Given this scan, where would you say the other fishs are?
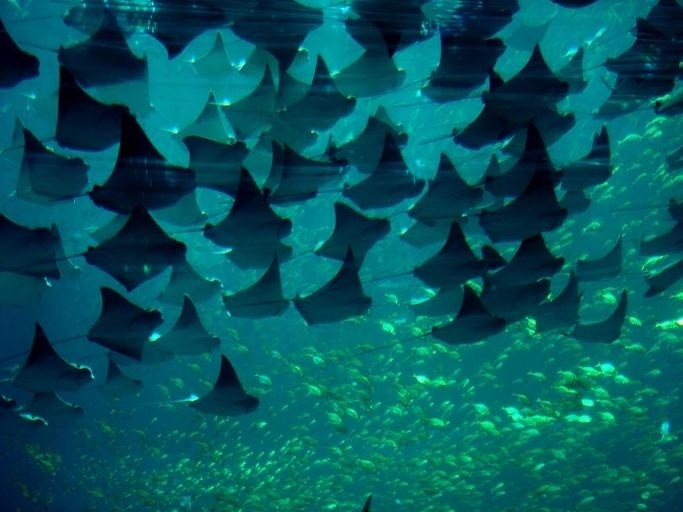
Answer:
[0,0,683,512]
